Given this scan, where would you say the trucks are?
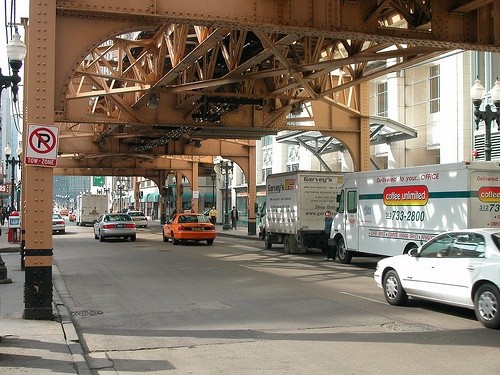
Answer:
[253,170,355,254]
[75,194,108,226]
[329,161,500,264]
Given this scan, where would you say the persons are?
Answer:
[0,205,16,225]
[210,207,217,225]
[324,211,336,260]
[231,206,238,230]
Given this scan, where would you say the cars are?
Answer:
[126,211,147,228]
[69,210,76,222]
[92,213,136,241]
[60,209,68,216]
[52,214,65,234]
[162,209,216,245]
[374,227,499,330]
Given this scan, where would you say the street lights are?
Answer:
[471,74,500,162]
[97,183,111,195]
[4,145,22,212]
[0,26,27,103]
[117,180,125,213]
[219,157,235,230]
[211,173,216,206]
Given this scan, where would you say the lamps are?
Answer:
[194,141,201,148]
[290,107,303,115]
[99,138,106,147]
[146,95,159,110]
